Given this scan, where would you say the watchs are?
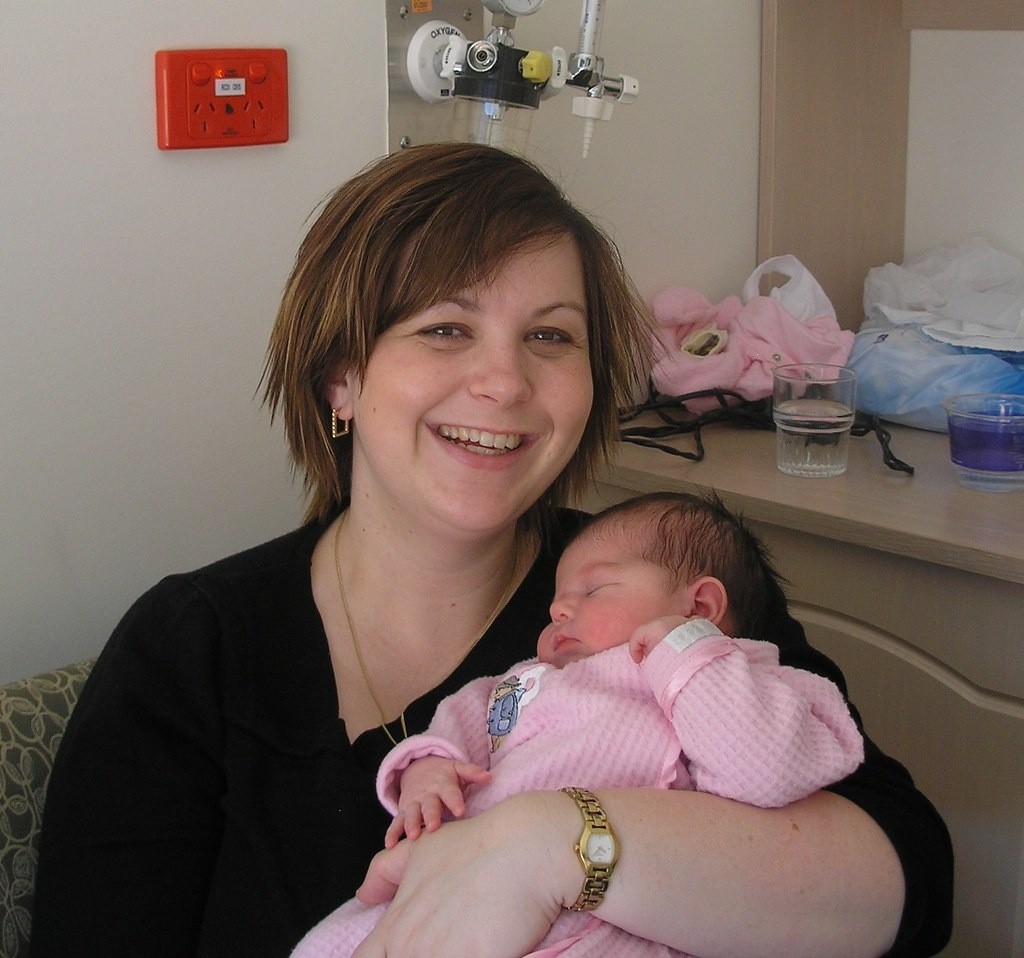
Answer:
[560,787,619,914]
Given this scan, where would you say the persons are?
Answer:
[31,140,957,958]
[290,491,869,958]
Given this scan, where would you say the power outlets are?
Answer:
[154,47,290,151]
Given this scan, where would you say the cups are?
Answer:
[772,363,857,478]
[940,393,1024,492]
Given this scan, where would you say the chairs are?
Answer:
[0,656,96,958]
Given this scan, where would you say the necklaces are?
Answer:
[334,505,519,746]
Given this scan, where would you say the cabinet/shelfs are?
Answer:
[568,398,1024,958]
